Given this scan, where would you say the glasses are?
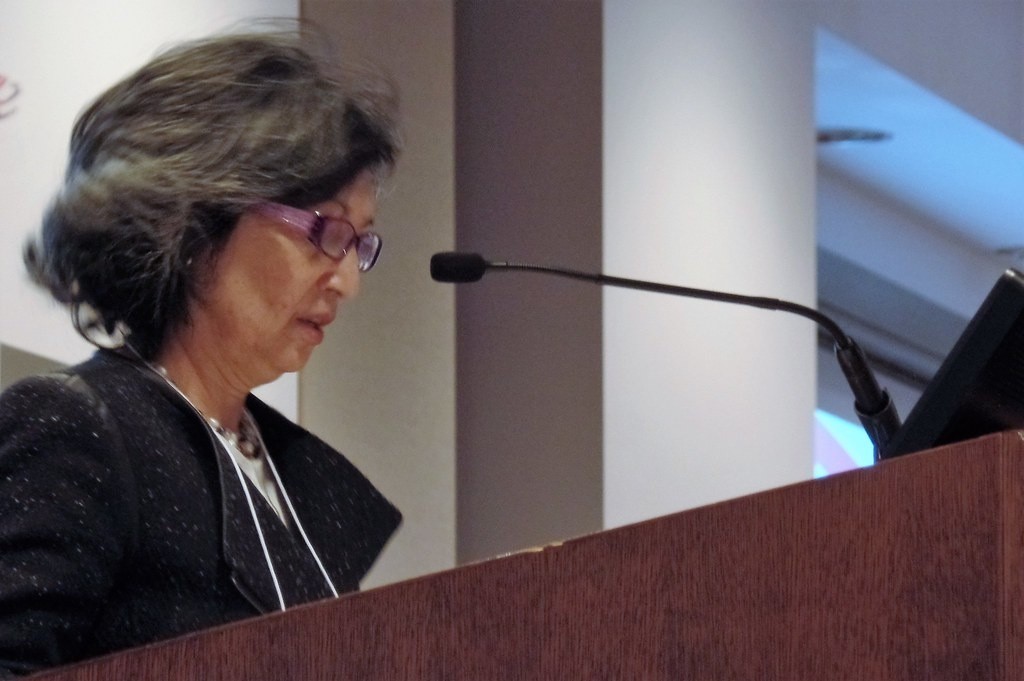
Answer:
[241,197,382,272]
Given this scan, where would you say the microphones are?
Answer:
[431,251,902,463]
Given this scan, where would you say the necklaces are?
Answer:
[161,362,258,457]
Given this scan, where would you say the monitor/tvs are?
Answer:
[889,269,1024,456]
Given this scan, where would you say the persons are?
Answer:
[0,19,409,681]
